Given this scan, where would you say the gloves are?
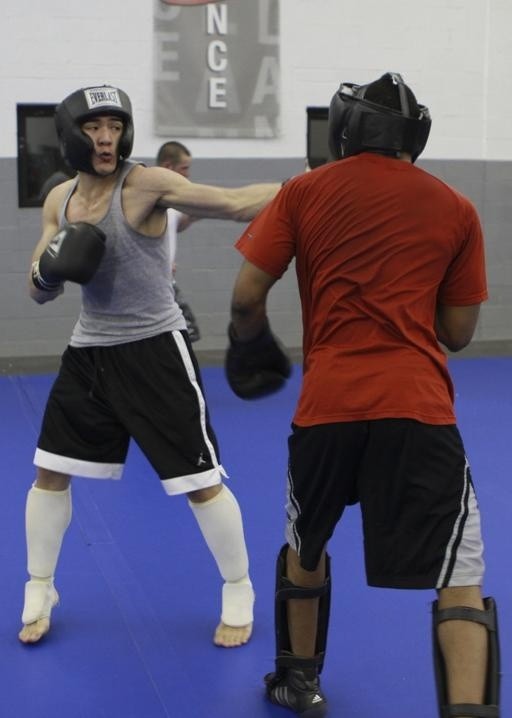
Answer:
[225,316,292,401]
[32,221,107,291]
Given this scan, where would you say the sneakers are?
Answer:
[263,670,330,718]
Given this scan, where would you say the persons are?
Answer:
[155,142,201,343]
[16,86,282,648]
[38,164,75,200]
[225,71,502,717]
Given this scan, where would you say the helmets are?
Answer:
[328,72,432,162]
[56,85,133,175]
